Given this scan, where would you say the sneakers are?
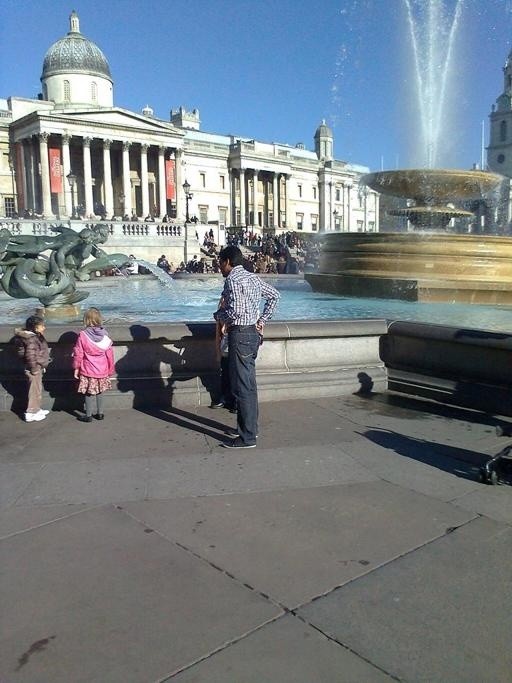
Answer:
[211,402,225,408]
[227,429,259,437]
[25,409,50,422]
[95,414,104,419]
[223,437,257,449]
[78,416,91,422]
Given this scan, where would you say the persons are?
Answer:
[13,316,50,422]
[10,207,200,223]
[115,229,319,276]
[72,307,116,422]
[210,298,233,409]
[213,245,281,448]
[2,223,112,292]
